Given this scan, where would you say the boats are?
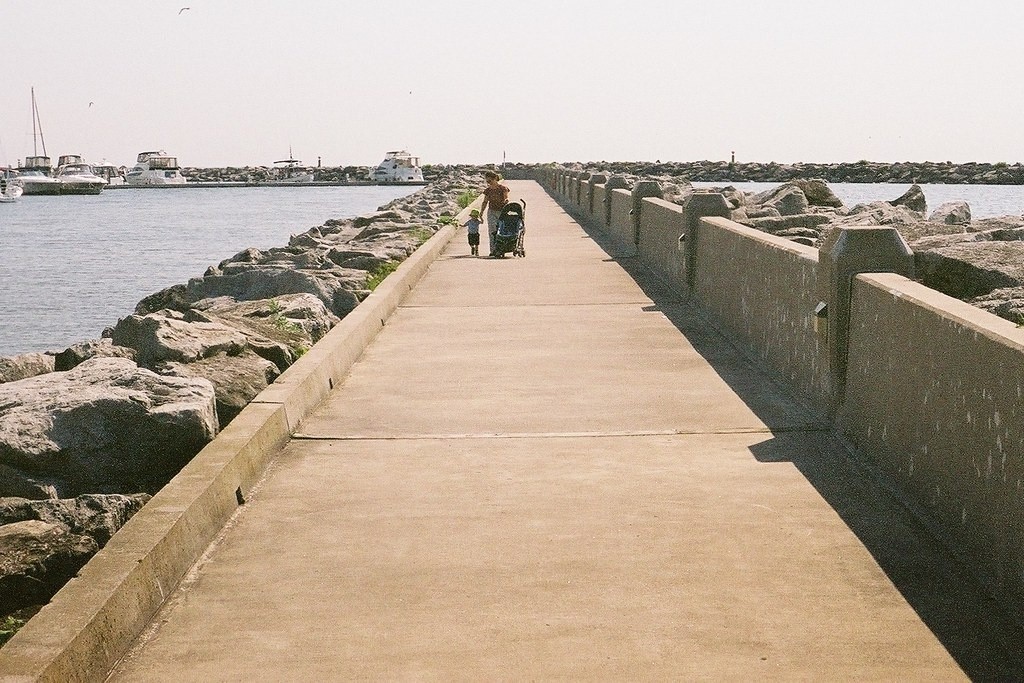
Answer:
[372,149,424,185]
[0,150,187,202]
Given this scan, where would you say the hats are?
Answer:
[469,208,480,218]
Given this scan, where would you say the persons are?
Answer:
[480,170,510,257]
[460,209,484,257]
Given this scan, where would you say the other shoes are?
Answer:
[471,247,479,256]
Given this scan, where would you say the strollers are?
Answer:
[491,199,526,259]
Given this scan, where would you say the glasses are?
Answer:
[486,179,491,182]
